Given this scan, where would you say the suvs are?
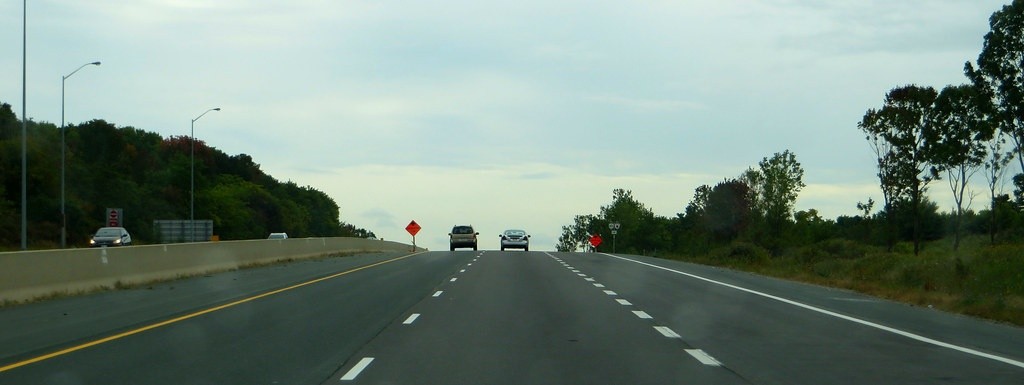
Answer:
[448,225,479,252]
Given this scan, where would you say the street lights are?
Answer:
[60,61,102,248]
[190,108,220,242]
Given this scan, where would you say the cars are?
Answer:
[499,230,531,251]
[88,226,132,246]
[268,233,288,238]
